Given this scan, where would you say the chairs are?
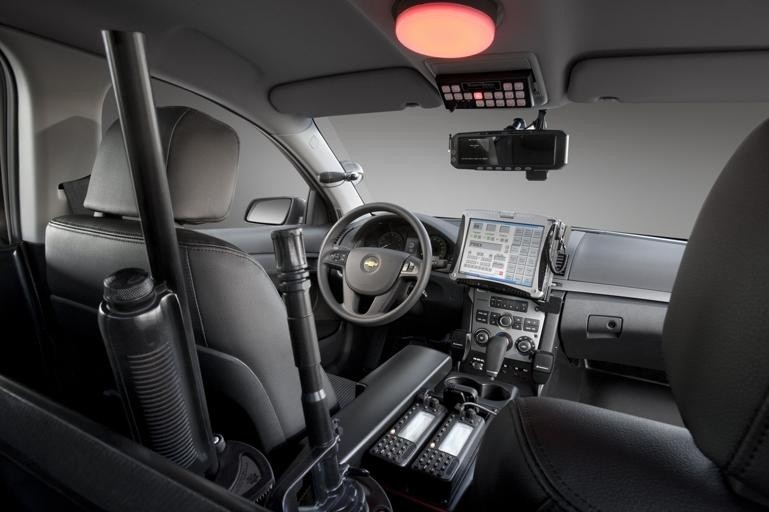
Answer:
[472,117,768,511]
[44,106,339,452]
